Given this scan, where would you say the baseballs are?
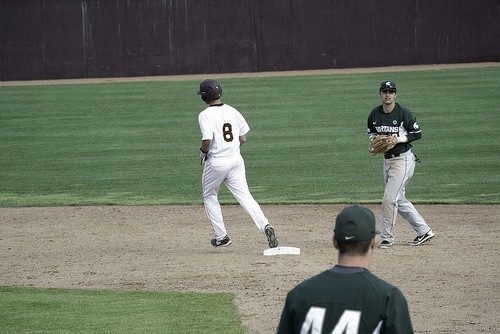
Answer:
[370,147,375,152]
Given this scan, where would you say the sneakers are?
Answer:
[380,238,395,246]
[265,224,279,248]
[408,229,436,246]
[210,236,232,248]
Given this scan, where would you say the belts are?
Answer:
[384,153,399,160]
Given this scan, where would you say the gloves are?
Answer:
[199,148,208,160]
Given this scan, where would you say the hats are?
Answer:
[379,81,397,93]
[336,203,382,242]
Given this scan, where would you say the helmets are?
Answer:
[198,79,221,103]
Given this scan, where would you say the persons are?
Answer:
[367,80,435,248]
[197,79,280,248]
[276,204,414,334]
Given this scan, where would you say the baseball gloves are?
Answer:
[371,134,398,154]
